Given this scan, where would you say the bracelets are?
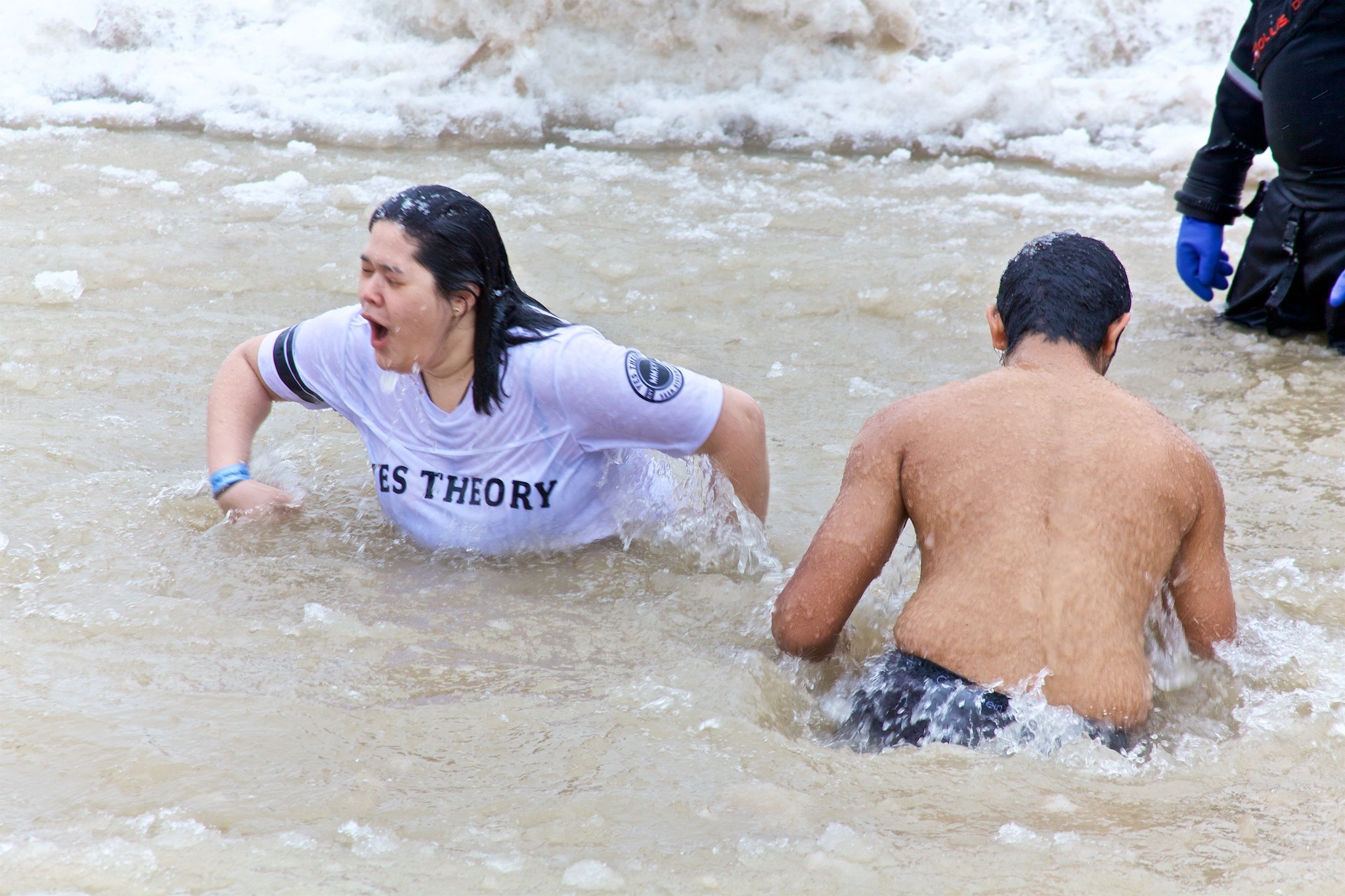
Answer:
[208,464,251,498]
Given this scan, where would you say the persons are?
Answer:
[770,230,1236,741]
[207,184,771,553]
[1174,0,1345,358]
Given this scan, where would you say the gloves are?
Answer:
[1176,215,1234,302]
[1330,270,1345,307]
